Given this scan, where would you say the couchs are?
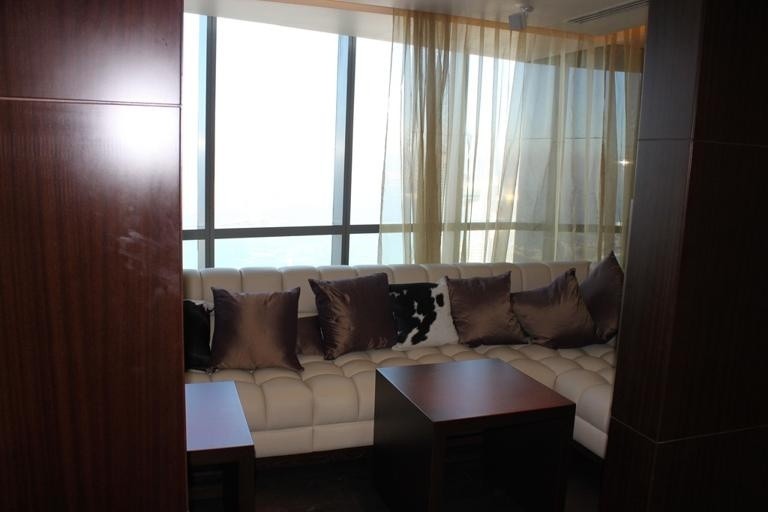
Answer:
[184,259,614,459]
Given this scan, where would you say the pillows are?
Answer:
[387,274,457,349]
[210,285,306,374]
[581,250,626,340]
[502,268,595,348]
[305,273,395,360]
[441,268,529,346]
[183,298,215,374]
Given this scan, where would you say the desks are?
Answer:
[184,379,254,510]
